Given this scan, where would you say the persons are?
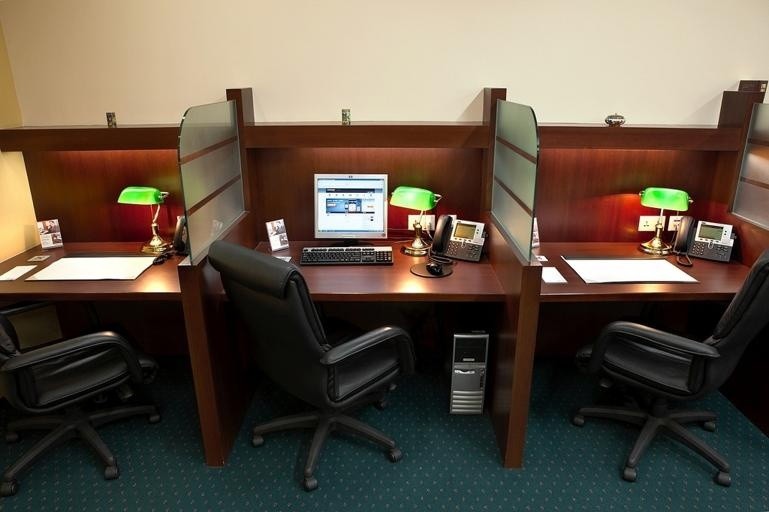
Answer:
[270,220,286,236]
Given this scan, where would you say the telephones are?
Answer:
[672,216,736,263]
[430,215,486,262]
[173,216,189,255]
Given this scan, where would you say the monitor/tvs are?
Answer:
[314,173,388,247]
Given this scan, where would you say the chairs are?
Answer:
[571,248,768,485]
[0,300,164,498]
[208,242,417,492]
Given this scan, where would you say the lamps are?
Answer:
[117,186,173,255]
[638,185,694,257]
[390,185,442,258]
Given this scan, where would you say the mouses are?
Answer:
[426,262,442,275]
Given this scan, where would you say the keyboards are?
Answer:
[300,247,393,265]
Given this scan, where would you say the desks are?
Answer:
[0,89,253,467]
[177,88,545,469]
[483,80,768,469]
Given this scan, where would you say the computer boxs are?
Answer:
[449,331,490,415]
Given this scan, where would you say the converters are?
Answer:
[426,216,431,232]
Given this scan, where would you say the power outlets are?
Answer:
[407,214,435,232]
[638,215,666,233]
